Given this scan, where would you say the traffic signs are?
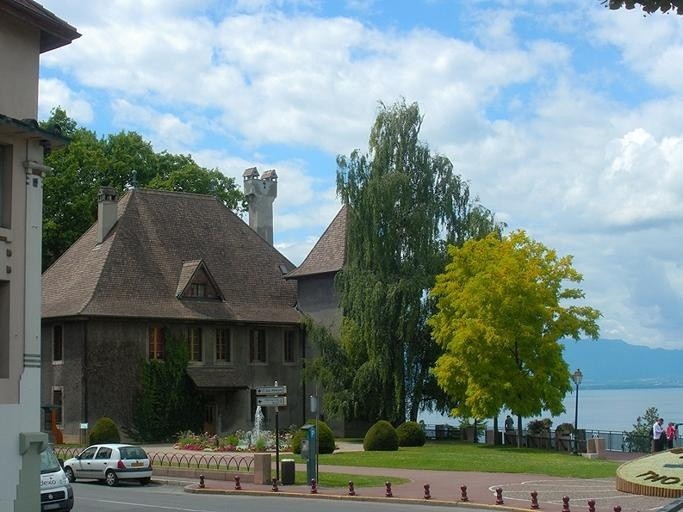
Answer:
[256,396,288,406]
[255,386,288,396]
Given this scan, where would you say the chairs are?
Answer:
[129,451,136,456]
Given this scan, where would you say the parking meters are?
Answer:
[301,425,317,485]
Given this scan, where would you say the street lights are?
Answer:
[571,369,582,455]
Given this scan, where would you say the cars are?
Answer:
[62,444,153,487]
[39,443,74,512]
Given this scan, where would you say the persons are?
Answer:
[665,423,679,449]
[649,427,663,454]
[654,417,665,451]
[505,415,515,432]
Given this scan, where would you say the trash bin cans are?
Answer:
[281,459,295,486]
[79,423,89,448]
